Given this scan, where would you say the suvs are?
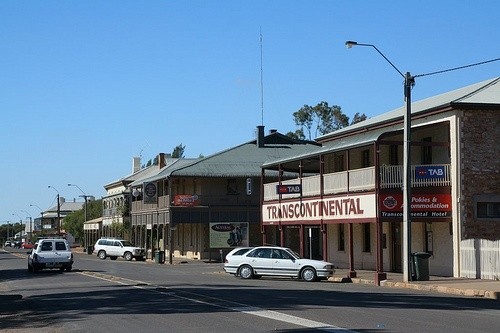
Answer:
[93,238,145,261]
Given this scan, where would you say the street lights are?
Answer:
[30,205,43,236]
[346,40,411,282]
[48,185,61,236]
[12,213,22,241]
[68,184,87,249]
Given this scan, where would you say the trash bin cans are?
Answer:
[154,251,164,263]
[411,252,431,281]
[87,246,93,254]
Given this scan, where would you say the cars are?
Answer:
[26,239,74,274]
[223,246,335,282]
[5,241,10,247]
